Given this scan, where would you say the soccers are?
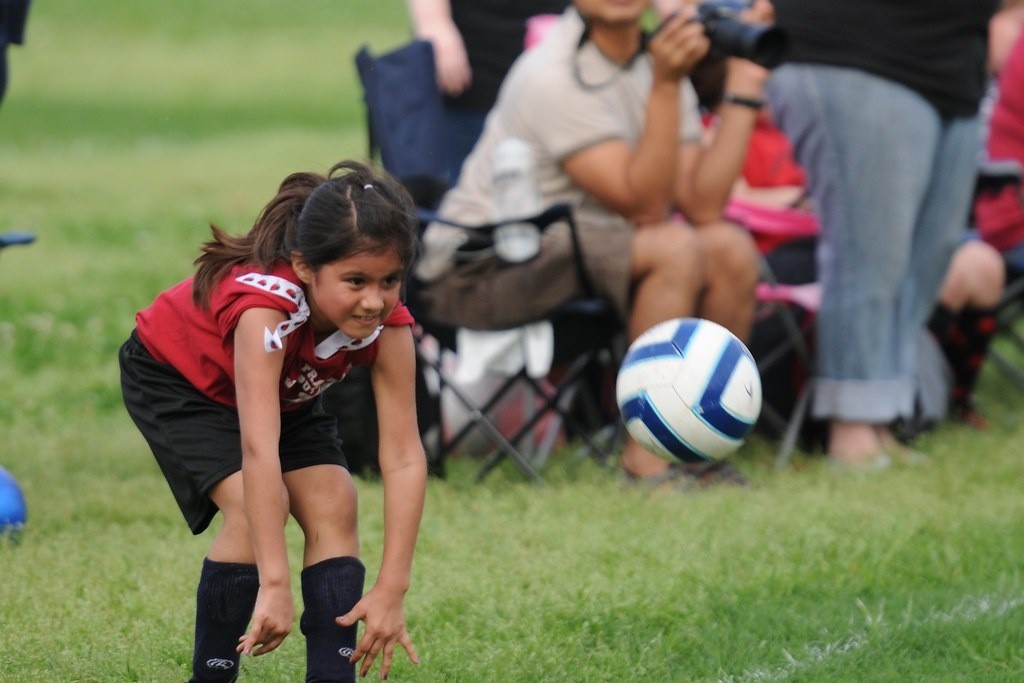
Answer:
[616,315,765,468]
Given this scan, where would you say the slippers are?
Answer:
[624,452,750,498]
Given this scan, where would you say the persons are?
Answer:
[414,0,1024,485]
[119,160,428,683]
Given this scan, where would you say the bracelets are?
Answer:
[723,93,768,113]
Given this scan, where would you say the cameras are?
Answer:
[688,3,789,70]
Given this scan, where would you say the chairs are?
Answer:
[353,35,1024,485]
